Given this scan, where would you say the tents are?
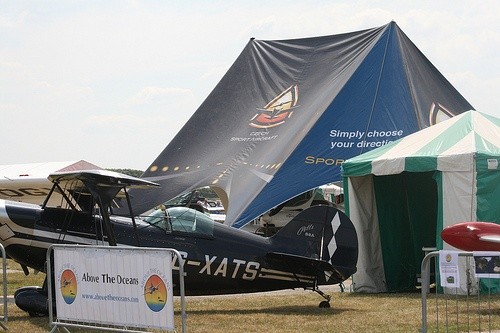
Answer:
[339,109,500,295]
[105,20,479,232]
[313,183,343,205]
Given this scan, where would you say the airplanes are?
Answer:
[0,170,358,318]
